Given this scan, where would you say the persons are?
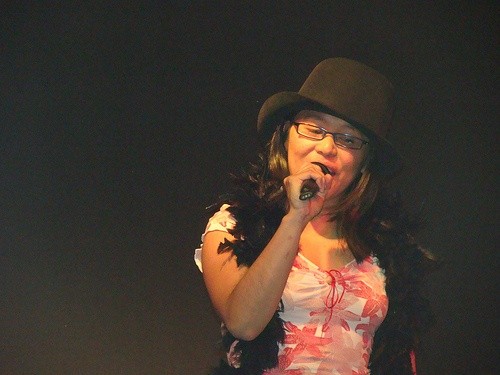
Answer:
[191,58,430,375]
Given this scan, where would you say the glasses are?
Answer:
[290,120,372,150]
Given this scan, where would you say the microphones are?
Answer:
[298,161,330,200]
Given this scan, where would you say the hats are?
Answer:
[256,54,402,180]
